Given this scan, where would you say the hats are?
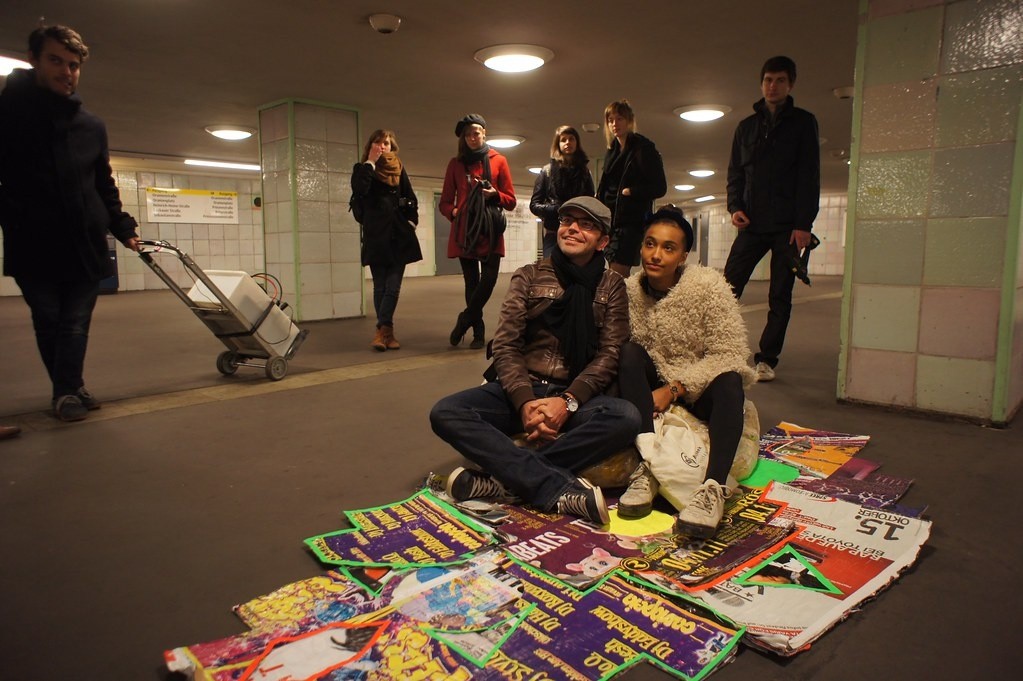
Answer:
[558,196,611,234]
[455,114,486,137]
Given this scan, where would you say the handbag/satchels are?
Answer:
[635,412,710,512]
[468,205,506,238]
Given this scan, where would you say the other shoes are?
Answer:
[470,328,485,348]
[450,312,471,346]
[0,425,21,437]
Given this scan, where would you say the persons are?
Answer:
[350,129,423,353]
[0,25,141,421]
[724,56,820,382]
[429,196,643,524]
[529,126,595,258]
[439,114,516,349]
[617,203,760,536]
[596,100,667,277]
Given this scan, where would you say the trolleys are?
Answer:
[133,238,310,381]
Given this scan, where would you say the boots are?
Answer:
[385,326,400,349]
[371,325,392,351]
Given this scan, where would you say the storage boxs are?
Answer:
[188,270,300,355]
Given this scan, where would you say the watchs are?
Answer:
[556,394,579,416]
[670,382,679,403]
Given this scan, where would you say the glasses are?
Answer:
[558,213,604,234]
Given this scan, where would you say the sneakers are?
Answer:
[447,467,519,503]
[617,462,659,517]
[51,394,88,421]
[557,477,611,525]
[677,478,733,538]
[755,362,775,381]
[74,386,101,409]
